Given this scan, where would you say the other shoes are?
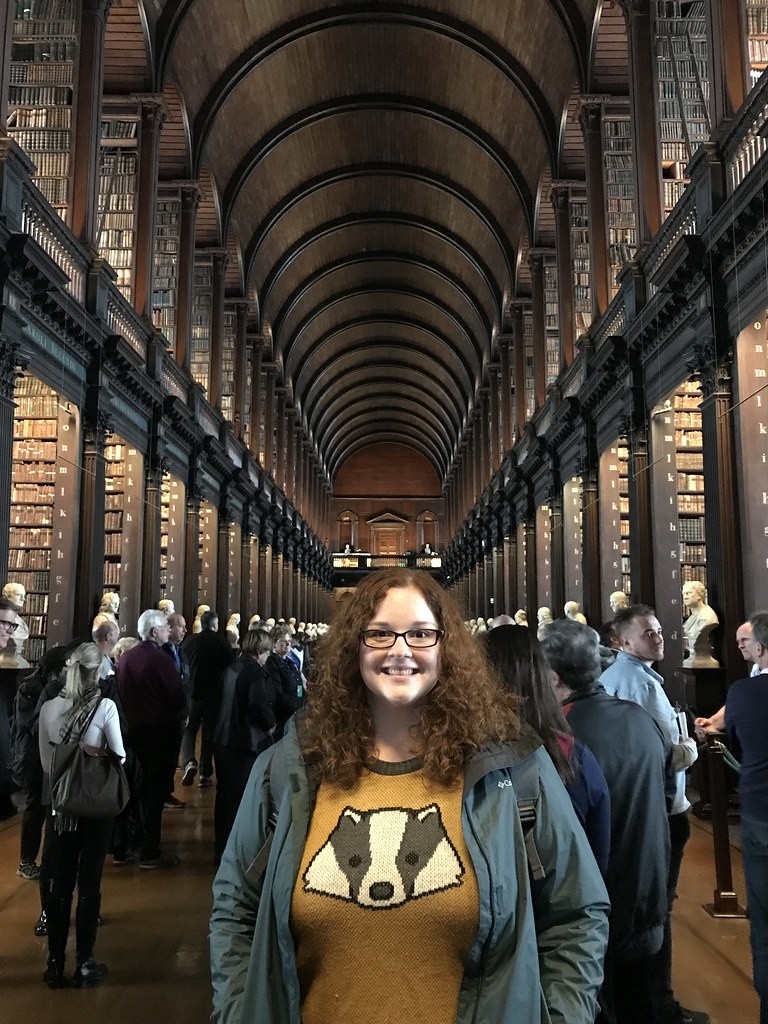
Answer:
[77,954,107,988]
[42,969,71,989]
[139,854,180,868]
[34,908,49,935]
[182,761,197,786]
[164,795,186,808]
[113,853,135,863]
[198,774,213,788]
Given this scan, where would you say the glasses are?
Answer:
[360,629,444,649]
[0,620,19,631]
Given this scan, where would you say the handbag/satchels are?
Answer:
[48,696,130,818]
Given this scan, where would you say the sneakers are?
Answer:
[16,861,40,878]
[680,1004,710,1024]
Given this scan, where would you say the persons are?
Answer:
[537,606,554,642]
[92,592,121,633]
[210,567,610,1024]
[476,604,768,1024]
[158,599,175,616]
[248,614,330,637]
[515,608,529,627]
[212,626,306,874]
[564,600,587,625]
[193,605,210,633]
[609,591,628,614]
[226,613,240,648]
[0,583,29,668]
[0,601,240,989]
[682,581,719,669]
[464,617,494,636]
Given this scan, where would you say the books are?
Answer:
[7,0,264,664]
[520,0,768,617]
